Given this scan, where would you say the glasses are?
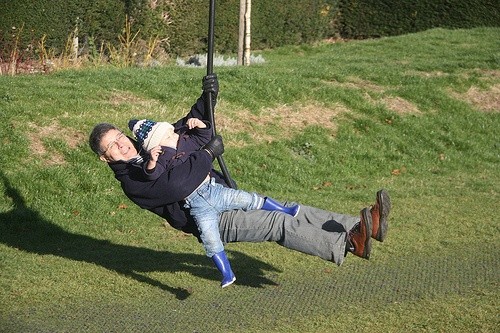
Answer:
[102,131,124,155]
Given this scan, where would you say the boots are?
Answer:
[261,196,300,218]
[212,250,236,288]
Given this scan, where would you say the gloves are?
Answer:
[202,135,224,158]
[201,73,219,108]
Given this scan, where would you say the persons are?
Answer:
[89,72,392,268]
[127,116,300,289]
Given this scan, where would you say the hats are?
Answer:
[129,118,175,155]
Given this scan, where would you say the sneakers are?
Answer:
[370,189,391,242]
[348,207,372,260]
